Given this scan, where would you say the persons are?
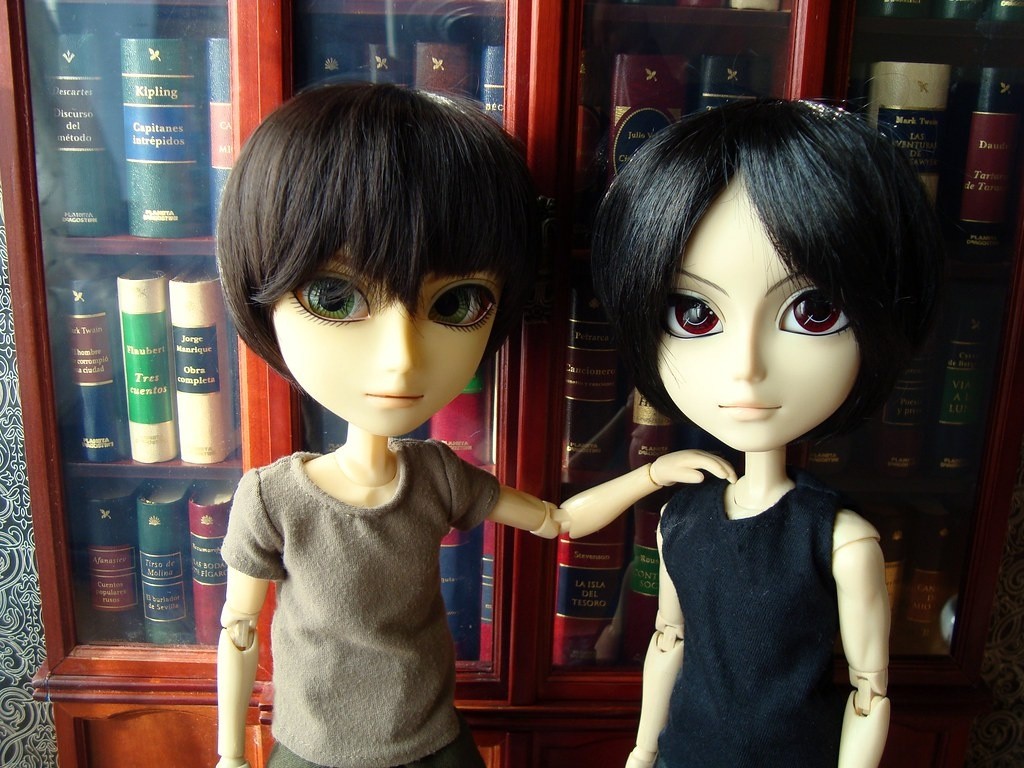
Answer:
[590,99,944,768]
[212,74,742,767]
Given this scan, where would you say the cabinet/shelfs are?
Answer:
[0,0,1024,768]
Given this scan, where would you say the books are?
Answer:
[18,0,1024,673]
